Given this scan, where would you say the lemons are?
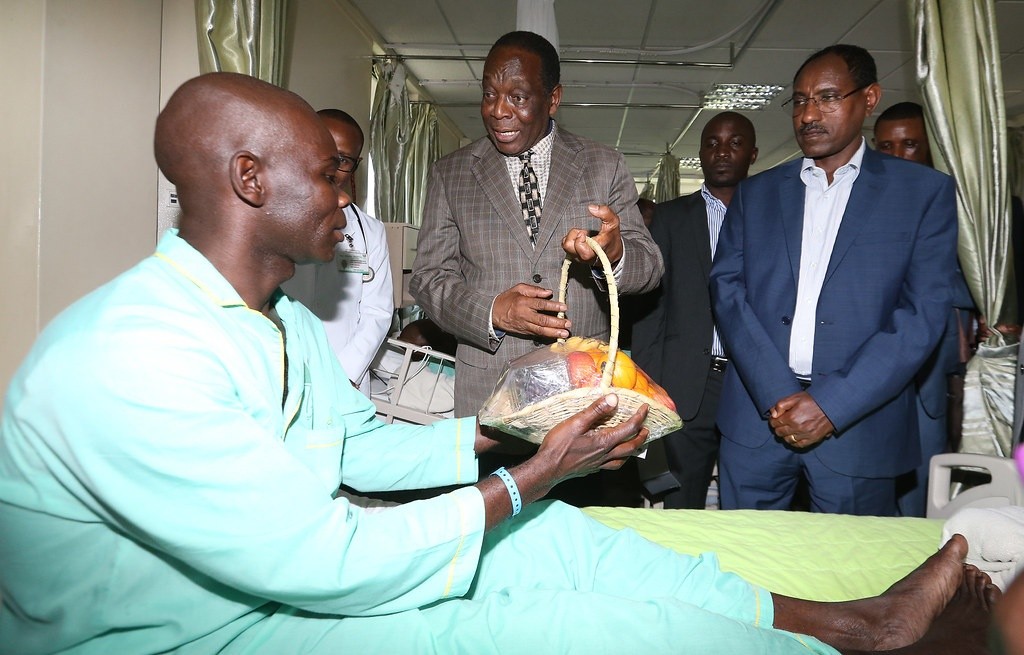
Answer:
[588,348,657,400]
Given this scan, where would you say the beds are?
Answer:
[578,454,1024,602]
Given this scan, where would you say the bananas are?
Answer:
[551,336,613,352]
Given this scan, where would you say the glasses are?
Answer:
[334,155,361,173]
[780,84,869,117]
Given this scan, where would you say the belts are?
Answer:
[710,356,728,372]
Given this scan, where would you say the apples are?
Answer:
[651,393,677,413]
[565,351,608,389]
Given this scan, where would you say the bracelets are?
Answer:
[489,466,522,517]
[489,293,501,343]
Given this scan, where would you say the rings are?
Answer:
[791,434,799,443]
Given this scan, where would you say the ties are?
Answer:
[518,150,542,251]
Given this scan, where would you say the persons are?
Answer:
[635,197,658,230]
[709,44,960,517]
[410,30,666,481]
[280,108,395,401]
[0,73,1024,654]
[370,319,443,393]
[630,110,759,508]
[871,101,980,517]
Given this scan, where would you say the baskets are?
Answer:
[477,233,683,459]
